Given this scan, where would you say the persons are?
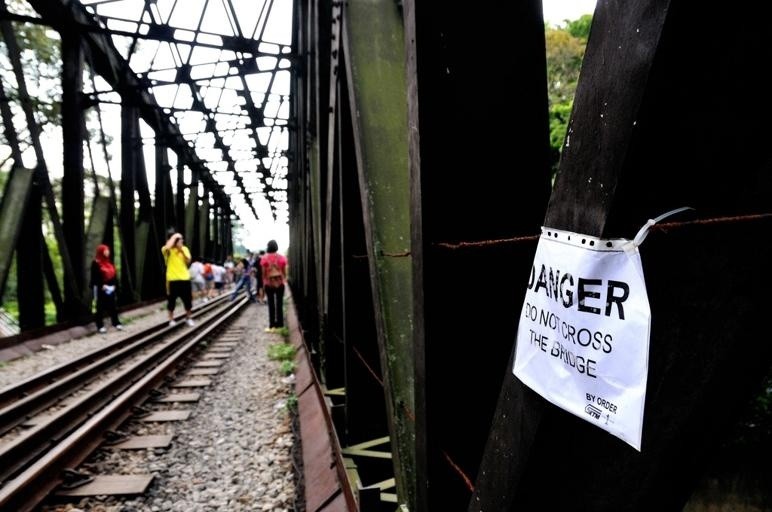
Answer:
[90,244,122,333]
[161,232,194,327]
[189,250,264,305]
[262,240,288,329]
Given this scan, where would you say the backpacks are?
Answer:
[264,254,282,288]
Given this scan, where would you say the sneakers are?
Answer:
[116,325,123,330]
[99,327,106,333]
[186,319,194,326]
[265,327,283,332]
[169,320,176,326]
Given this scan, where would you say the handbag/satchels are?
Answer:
[91,298,97,314]
